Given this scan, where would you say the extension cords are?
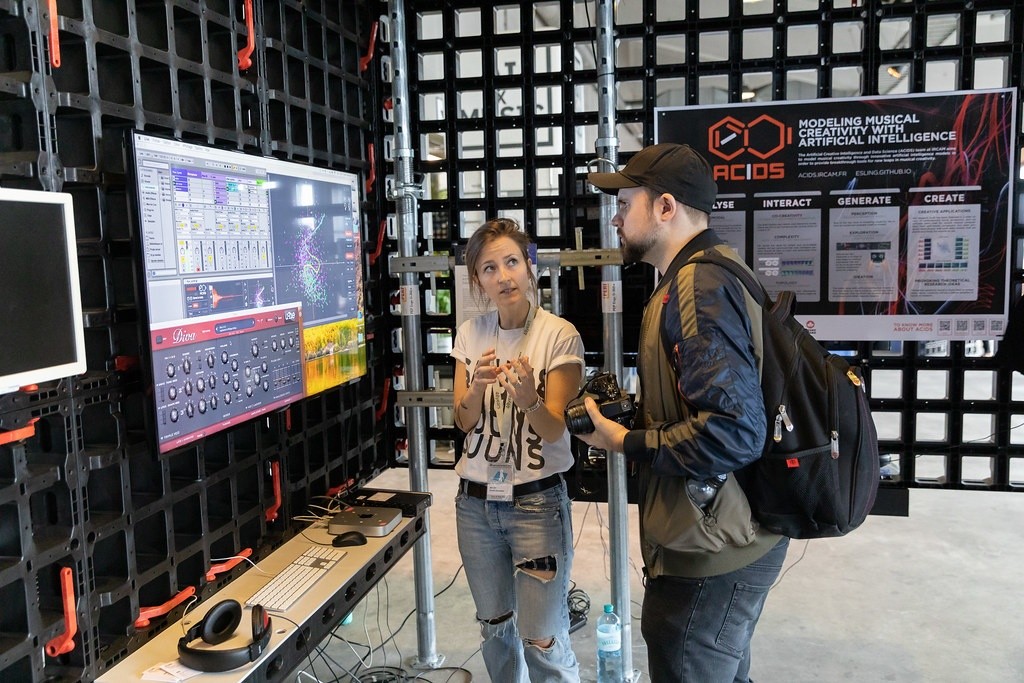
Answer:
[568,614,589,634]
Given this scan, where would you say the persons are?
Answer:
[574,141,795,683]
[450,218,586,683]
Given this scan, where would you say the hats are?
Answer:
[586,144,716,214]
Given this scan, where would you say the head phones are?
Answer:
[177,598,273,672]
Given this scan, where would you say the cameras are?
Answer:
[563,370,637,435]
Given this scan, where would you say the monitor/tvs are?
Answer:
[0,188,88,395]
[112,126,369,460]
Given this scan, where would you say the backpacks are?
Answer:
[678,255,879,540]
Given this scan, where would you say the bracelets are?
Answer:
[522,396,543,414]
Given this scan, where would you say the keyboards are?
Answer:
[243,546,348,614]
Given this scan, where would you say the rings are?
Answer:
[516,381,521,388]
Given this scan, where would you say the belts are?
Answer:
[459,477,560,498]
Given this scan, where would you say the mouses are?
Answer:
[332,531,367,547]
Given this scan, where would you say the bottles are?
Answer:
[686,474,727,508]
[596,604,624,683]
